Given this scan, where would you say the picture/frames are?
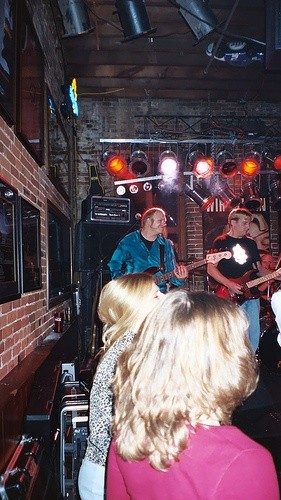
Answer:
[0,178,43,304]
[46,199,74,309]
[0,0,46,169]
[46,84,72,205]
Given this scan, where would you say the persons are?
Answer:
[79,207,281,500]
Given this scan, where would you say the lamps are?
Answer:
[187,150,214,178]
[239,152,262,179]
[56,0,221,42]
[183,173,281,211]
[215,151,239,178]
[129,145,151,177]
[158,149,179,174]
[265,155,281,170]
[104,146,127,176]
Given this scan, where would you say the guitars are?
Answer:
[143,247,233,287]
[221,267,281,300]
